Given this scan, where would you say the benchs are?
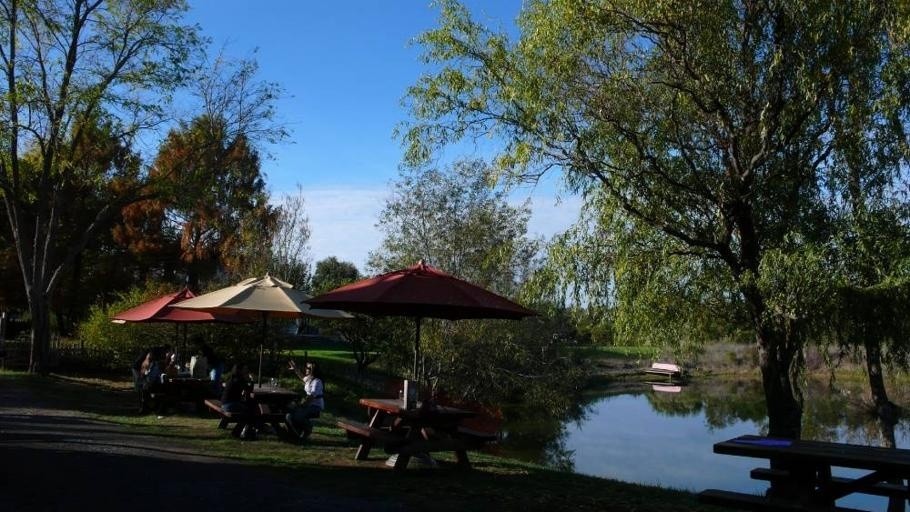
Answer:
[150,392,167,416]
[281,408,320,444]
[336,419,409,472]
[427,431,498,471]
[205,399,256,441]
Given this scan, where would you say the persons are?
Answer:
[221,361,259,441]
[285,359,324,442]
[133,344,208,415]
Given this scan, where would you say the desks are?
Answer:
[218,383,299,443]
[168,377,210,414]
[354,398,480,473]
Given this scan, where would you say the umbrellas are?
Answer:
[169,270,356,388]
[109,284,241,347]
[301,257,543,380]
